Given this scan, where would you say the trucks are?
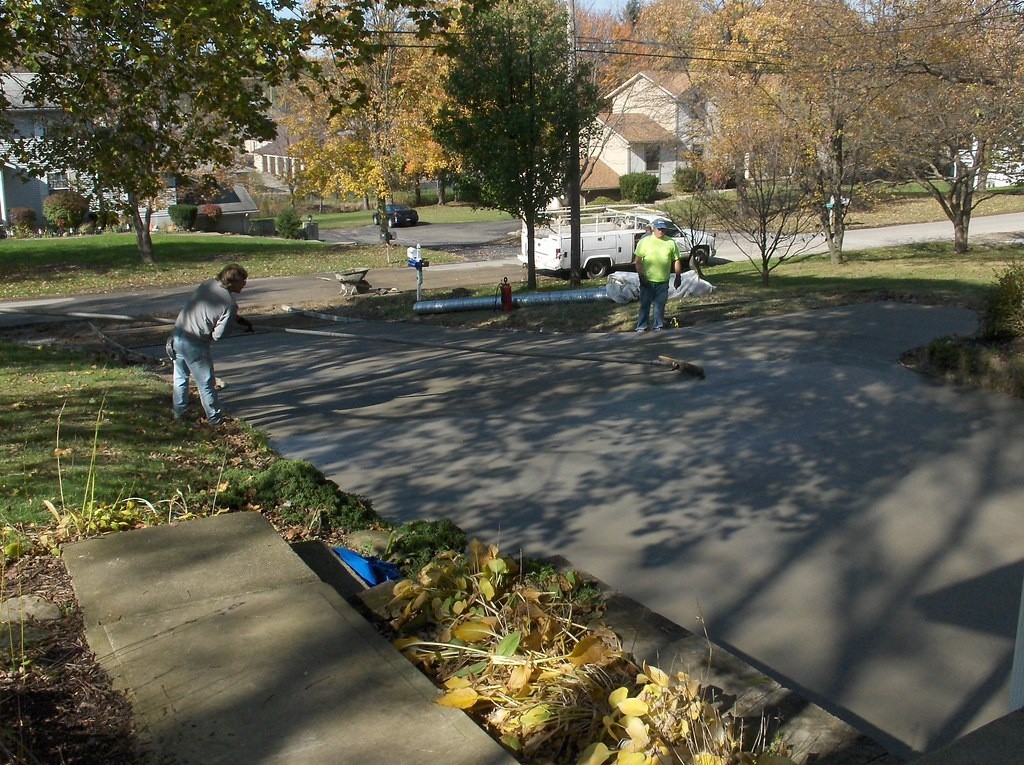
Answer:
[517,205,716,278]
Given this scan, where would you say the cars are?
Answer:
[372,204,419,228]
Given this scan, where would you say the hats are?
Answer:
[645,224,652,230]
[653,218,667,229]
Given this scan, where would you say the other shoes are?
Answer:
[653,328,662,331]
[637,329,645,332]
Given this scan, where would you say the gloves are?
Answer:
[674,275,681,289]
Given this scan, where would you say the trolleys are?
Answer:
[316,268,372,298]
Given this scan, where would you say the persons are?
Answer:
[636,218,681,332]
[152,225,158,231]
[172,263,247,425]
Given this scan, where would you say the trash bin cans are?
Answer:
[303,214,319,240]
